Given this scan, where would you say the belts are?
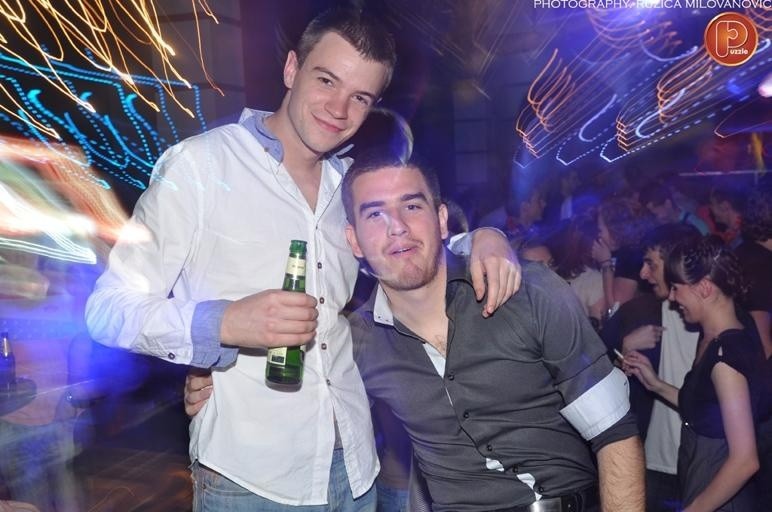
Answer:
[495,491,587,512]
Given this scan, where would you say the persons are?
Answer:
[336,102,414,512]
[182,144,650,511]
[1,236,95,511]
[82,0,400,512]
[498,160,771,511]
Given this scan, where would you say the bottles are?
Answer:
[265,238,312,388]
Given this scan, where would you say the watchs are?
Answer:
[63,389,82,410]
